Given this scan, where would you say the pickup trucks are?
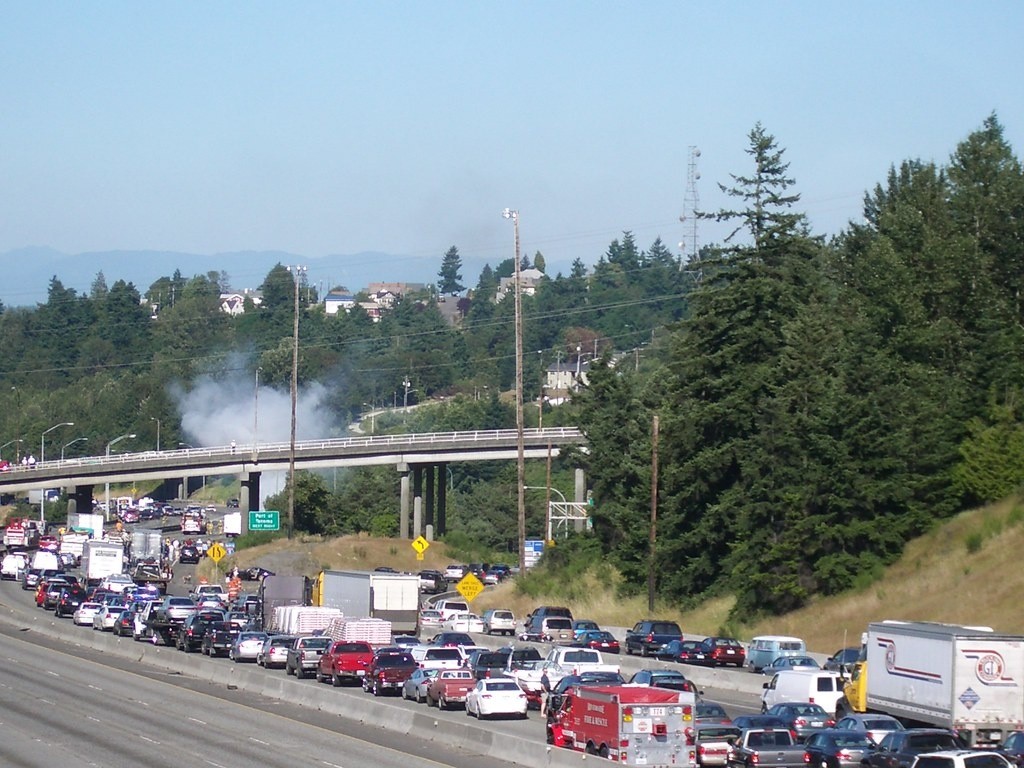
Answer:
[286,635,417,697]
[545,646,620,674]
[727,727,810,768]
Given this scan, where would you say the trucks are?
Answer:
[179,512,206,535]
[77,529,175,596]
[3,513,105,548]
[309,568,421,637]
[840,620,1024,748]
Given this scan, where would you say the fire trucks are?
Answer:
[546,682,700,768]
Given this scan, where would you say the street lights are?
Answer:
[524,486,567,539]
[41,418,205,521]
[363,403,374,436]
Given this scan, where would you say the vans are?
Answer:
[525,606,575,634]
[762,670,849,716]
[524,616,574,646]
[483,609,517,636]
[747,636,805,672]
[428,600,470,618]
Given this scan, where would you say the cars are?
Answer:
[0,547,297,667]
[415,595,745,695]
[122,498,238,522]
[697,702,1024,768]
[761,645,862,677]
[378,562,510,593]
[400,632,542,719]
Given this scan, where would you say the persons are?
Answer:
[160,513,168,527]
[231,439,236,455]
[206,520,214,535]
[21,456,27,470]
[162,536,224,567]
[540,667,550,718]
[115,521,122,532]
[58,526,67,535]
[217,519,223,535]
[28,454,36,469]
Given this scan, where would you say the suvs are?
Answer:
[624,619,683,657]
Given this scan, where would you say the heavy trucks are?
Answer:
[250,574,397,652]
